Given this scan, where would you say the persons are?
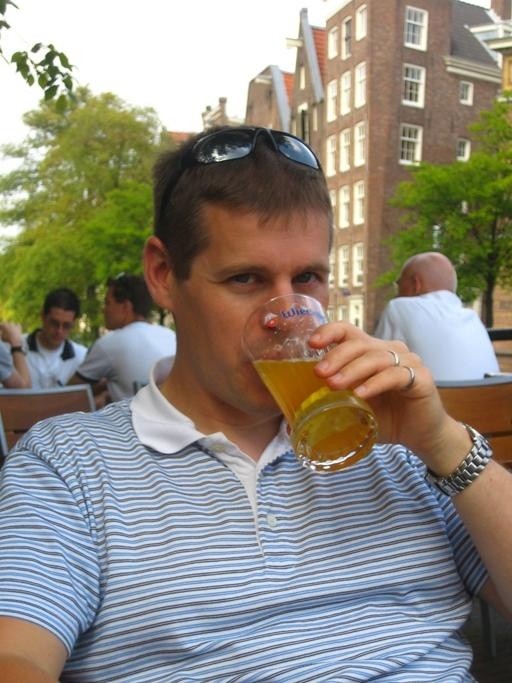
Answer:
[17,286,113,410]
[0,122,512,683]
[64,272,181,410]
[0,317,34,389]
[369,249,502,387]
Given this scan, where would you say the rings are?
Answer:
[418,421,496,498]
[383,348,401,365]
[400,364,416,391]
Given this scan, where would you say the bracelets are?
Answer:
[11,345,28,356]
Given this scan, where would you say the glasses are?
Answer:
[155,125,321,245]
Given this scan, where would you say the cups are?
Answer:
[240,294,379,476]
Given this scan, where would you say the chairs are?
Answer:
[1,384,95,459]
